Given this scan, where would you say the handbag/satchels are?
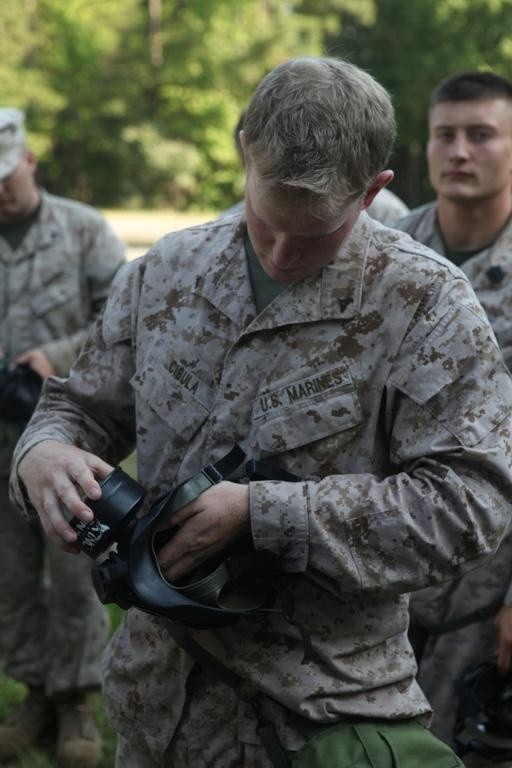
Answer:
[281,723,462,766]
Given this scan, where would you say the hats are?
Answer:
[0,108,25,182]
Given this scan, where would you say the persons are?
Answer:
[0,56,511,766]
[0,105,130,768]
[373,66,512,766]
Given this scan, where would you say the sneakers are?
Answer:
[0,687,101,768]
